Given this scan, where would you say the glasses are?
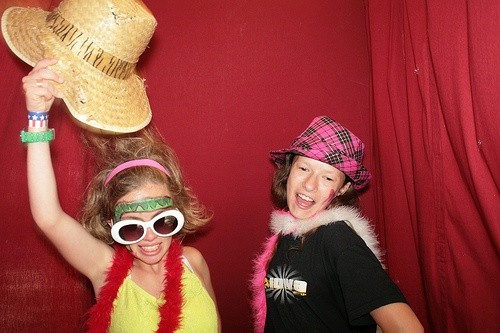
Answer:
[111,210,184,244]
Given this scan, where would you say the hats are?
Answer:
[270,116,371,189]
[2,0,156,133]
